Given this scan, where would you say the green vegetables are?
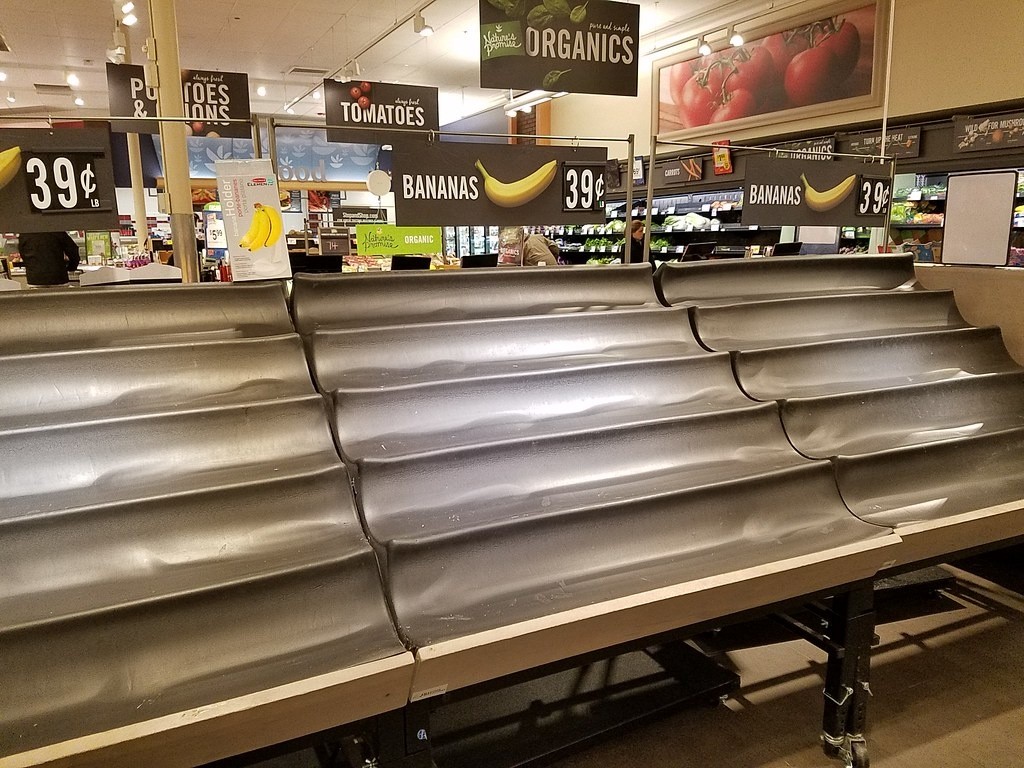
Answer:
[583,215,679,264]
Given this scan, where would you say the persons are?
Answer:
[523,234,560,265]
[17,231,81,286]
[621,220,658,272]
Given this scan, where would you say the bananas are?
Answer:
[475,158,559,208]
[799,172,856,212]
[239,203,282,252]
[0,147,22,190]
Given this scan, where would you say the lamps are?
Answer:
[64,69,80,86]
[7,90,16,102]
[308,83,321,99]
[112,0,138,27]
[696,38,714,56]
[286,105,297,115]
[335,66,353,83]
[412,9,436,37]
[727,24,746,48]
[72,93,88,107]
[503,48,653,121]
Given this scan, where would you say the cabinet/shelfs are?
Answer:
[0,179,1024,768]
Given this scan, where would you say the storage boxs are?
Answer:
[119,214,132,224]
[147,217,157,228]
[148,227,152,235]
[318,225,350,255]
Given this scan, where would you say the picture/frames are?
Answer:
[647,0,890,146]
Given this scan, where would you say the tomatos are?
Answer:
[351,82,371,108]
[668,16,862,130]
